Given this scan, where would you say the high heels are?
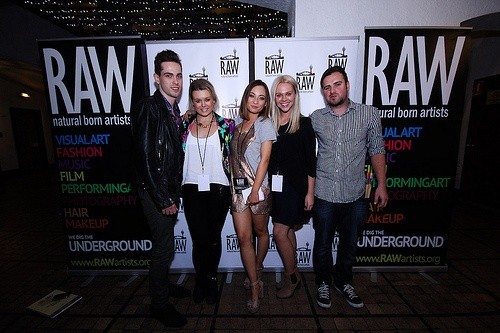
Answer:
[276,267,299,289]
[276,269,301,298]
[242,267,264,290]
[247,280,264,313]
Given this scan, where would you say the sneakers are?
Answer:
[169,283,191,298]
[317,280,331,308]
[149,304,187,327]
[334,283,363,308]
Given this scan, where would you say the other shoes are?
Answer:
[205,278,219,305]
[194,278,205,304]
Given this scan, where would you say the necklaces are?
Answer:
[195,119,214,128]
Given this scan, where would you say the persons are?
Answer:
[267,75,316,299]
[181,80,276,312]
[128,50,188,328]
[180,79,236,303]
[308,65,389,307]
